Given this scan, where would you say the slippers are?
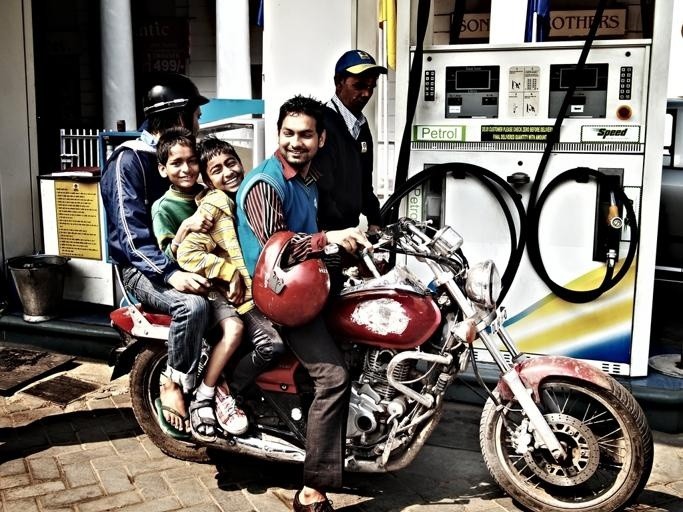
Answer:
[154,398,192,439]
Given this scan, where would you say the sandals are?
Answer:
[188,396,217,443]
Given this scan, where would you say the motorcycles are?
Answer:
[109,217,654,512]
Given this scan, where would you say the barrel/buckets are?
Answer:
[4,251,72,325]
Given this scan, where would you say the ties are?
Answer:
[354,123,361,135]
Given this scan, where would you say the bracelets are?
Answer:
[169,238,181,247]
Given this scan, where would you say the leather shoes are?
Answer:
[293,490,334,512]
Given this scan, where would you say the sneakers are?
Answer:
[213,386,248,435]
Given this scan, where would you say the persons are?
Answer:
[318,49,388,278]
[100,75,210,441]
[176,136,286,434]
[235,96,374,512]
[150,127,246,444]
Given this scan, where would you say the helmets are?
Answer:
[252,232,331,325]
[144,74,209,115]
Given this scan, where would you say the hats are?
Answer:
[334,50,387,74]
